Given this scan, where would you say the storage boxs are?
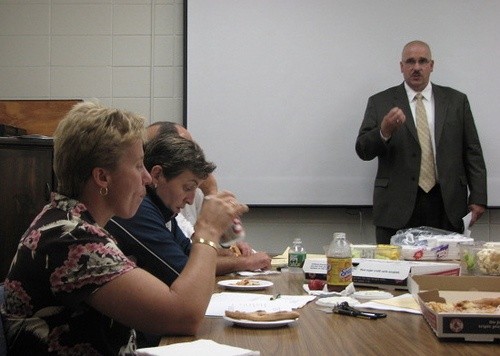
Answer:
[410,274,500,342]
[302,258,462,291]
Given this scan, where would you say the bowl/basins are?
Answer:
[351,244,402,260]
[456,240,500,277]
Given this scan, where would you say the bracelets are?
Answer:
[192,239,218,251]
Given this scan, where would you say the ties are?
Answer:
[414,93,436,194]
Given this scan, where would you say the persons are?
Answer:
[0,102,249,356]
[106,132,270,287]
[148,121,218,243]
[356,41,487,243]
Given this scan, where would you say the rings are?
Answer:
[397,119,399,123]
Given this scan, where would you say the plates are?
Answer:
[217,279,274,291]
[223,315,300,328]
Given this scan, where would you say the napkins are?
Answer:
[303,283,355,297]
[133,337,261,356]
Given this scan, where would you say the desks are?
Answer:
[158,252,500,356]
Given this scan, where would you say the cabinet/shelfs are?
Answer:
[0,137,57,283]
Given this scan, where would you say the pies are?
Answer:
[225,309,300,321]
[423,297,500,314]
[229,245,242,256]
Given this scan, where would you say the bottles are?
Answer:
[287,238,307,274]
[219,217,245,248]
[326,232,353,293]
